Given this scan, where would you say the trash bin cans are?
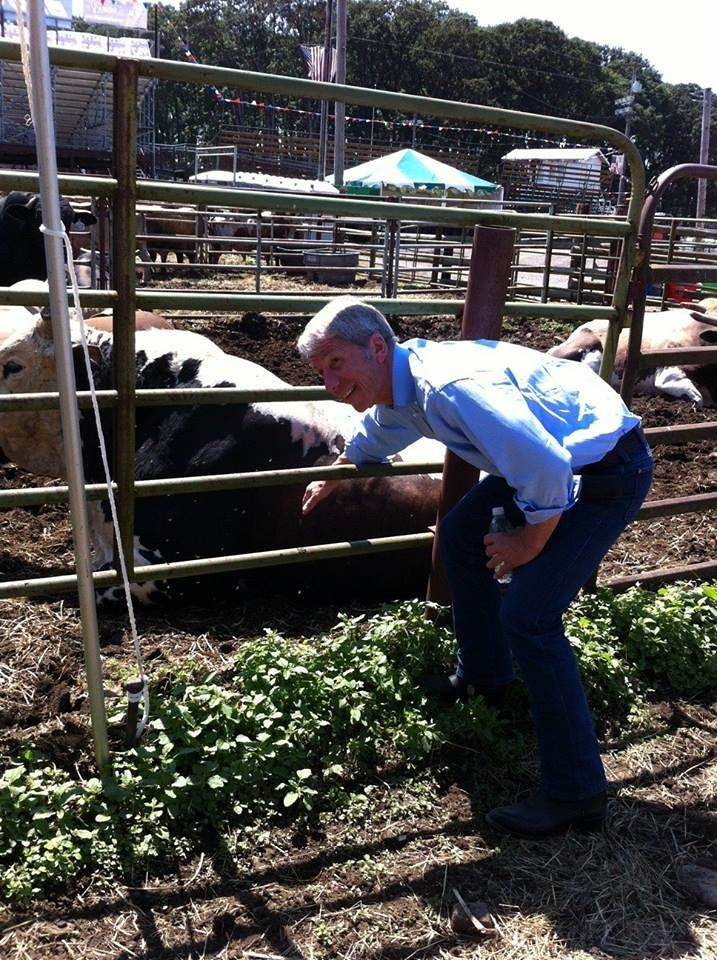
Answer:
[630,283,661,296]
[667,282,696,303]
[695,282,717,300]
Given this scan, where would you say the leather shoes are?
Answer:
[485,789,608,841]
[423,673,510,708]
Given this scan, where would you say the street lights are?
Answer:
[614,63,650,208]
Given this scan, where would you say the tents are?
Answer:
[319,148,504,216]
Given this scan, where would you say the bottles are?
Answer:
[489,507,515,584]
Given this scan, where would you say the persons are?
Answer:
[295,294,654,837]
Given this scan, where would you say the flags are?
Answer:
[302,43,338,83]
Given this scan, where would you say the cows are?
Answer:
[676,235,717,254]
[539,294,717,413]
[0,279,491,624]
[0,185,302,321]
